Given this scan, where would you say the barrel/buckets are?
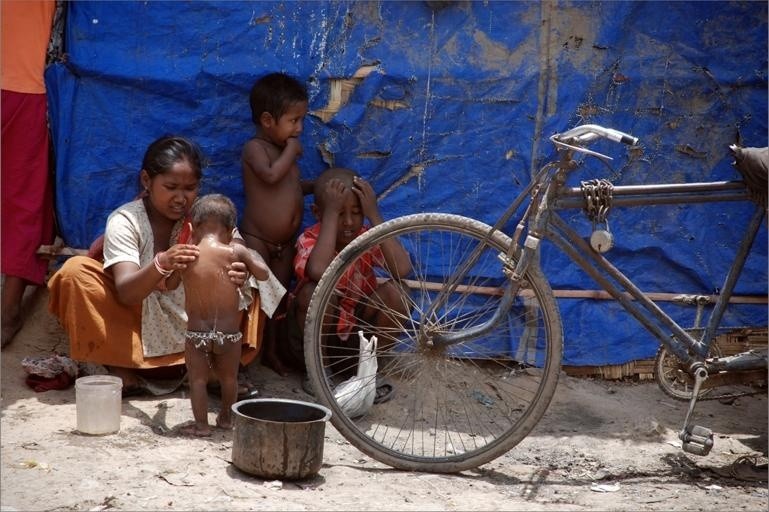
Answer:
[73,373,124,436]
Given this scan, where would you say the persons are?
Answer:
[267,167,415,404]
[240,73,318,378]
[2,1,54,352]
[158,193,270,437]
[46,133,291,403]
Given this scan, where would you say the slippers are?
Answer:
[374,372,395,405]
[302,368,333,396]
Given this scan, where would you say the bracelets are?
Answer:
[153,252,174,278]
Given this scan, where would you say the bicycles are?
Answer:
[298,122,768,475]
